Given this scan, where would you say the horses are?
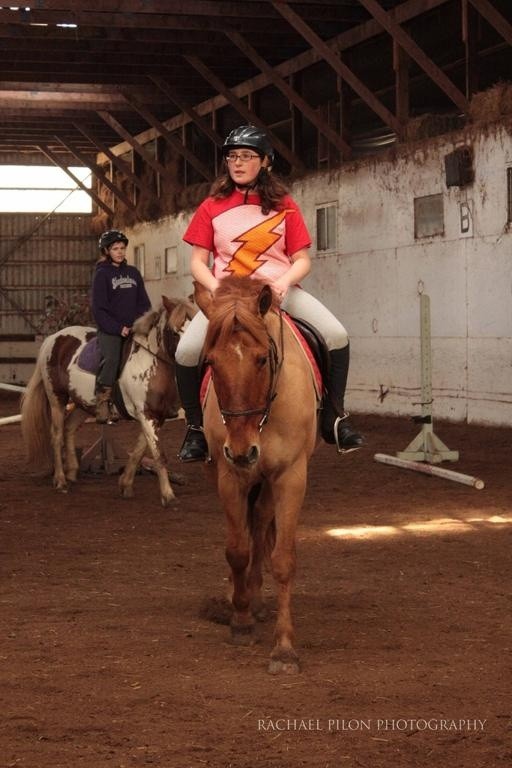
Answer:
[199,274,325,677]
[18,291,200,510]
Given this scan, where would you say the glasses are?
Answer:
[225,153,263,163]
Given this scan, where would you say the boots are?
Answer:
[319,340,367,449]
[94,384,118,424]
[175,360,207,462]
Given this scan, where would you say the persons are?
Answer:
[90,229,153,424]
[172,124,364,463]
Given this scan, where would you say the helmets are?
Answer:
[98,230,127,252]
[222,126,272,156]
